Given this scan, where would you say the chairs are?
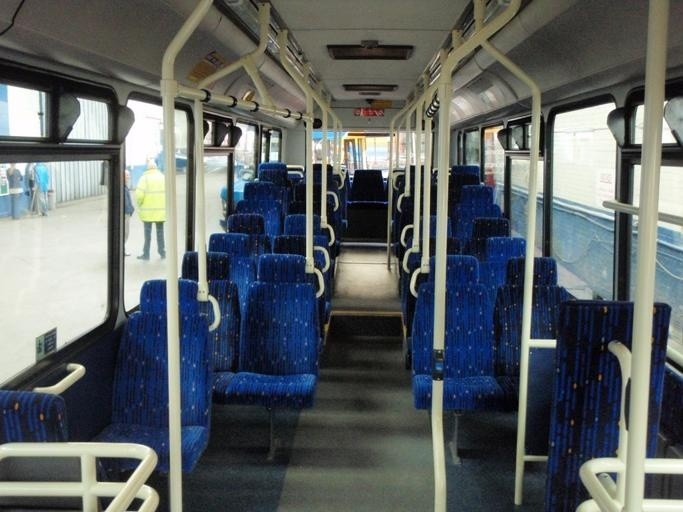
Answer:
[223,282,320,461]
[346,169,388,243]
[494,255,577,446]
[88,277,222,482]
[0,386,124,511]
[411,283,506,462]
[391,163,558,367]
[179,157,344,412]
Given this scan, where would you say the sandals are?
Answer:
[220,131,357,229]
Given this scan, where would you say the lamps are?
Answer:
[346,169,388,243]
[88,277,222,482]
[0,386,124,511]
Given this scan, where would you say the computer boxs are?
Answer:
[366,99,373,104]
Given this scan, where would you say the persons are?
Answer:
[25,162,36,213]
[122,169,135,258]
[34,160,49,217]
[5,163,23,220]
[485,160,497,205]
[133,158,166,260]
